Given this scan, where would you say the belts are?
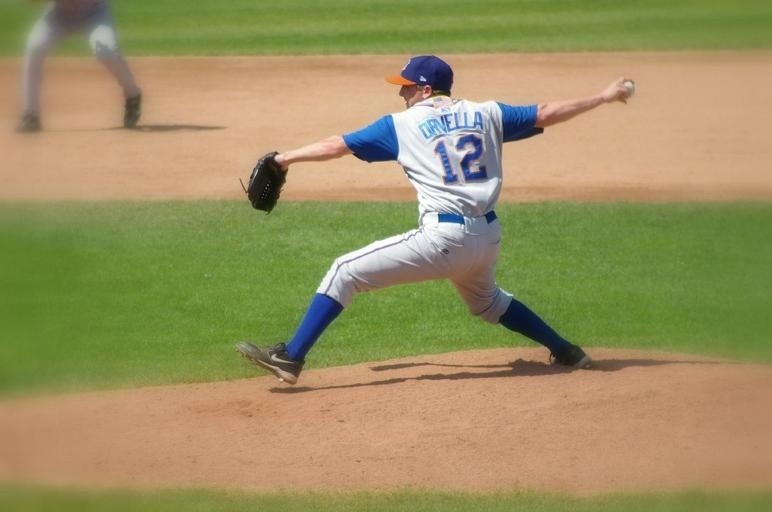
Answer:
[438,210,497,225]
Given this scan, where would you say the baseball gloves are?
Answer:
[247,151,288,211]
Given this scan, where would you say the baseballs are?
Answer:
[624,81,634,95]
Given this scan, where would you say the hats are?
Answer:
[386,56,452,90]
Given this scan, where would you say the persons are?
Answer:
[234,54,635,386]
[15,0,142,133]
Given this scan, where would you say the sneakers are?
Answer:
[233,339,305,384]
[550,345,591,368]
[15,113,41,133]
[124,94,140,127]
